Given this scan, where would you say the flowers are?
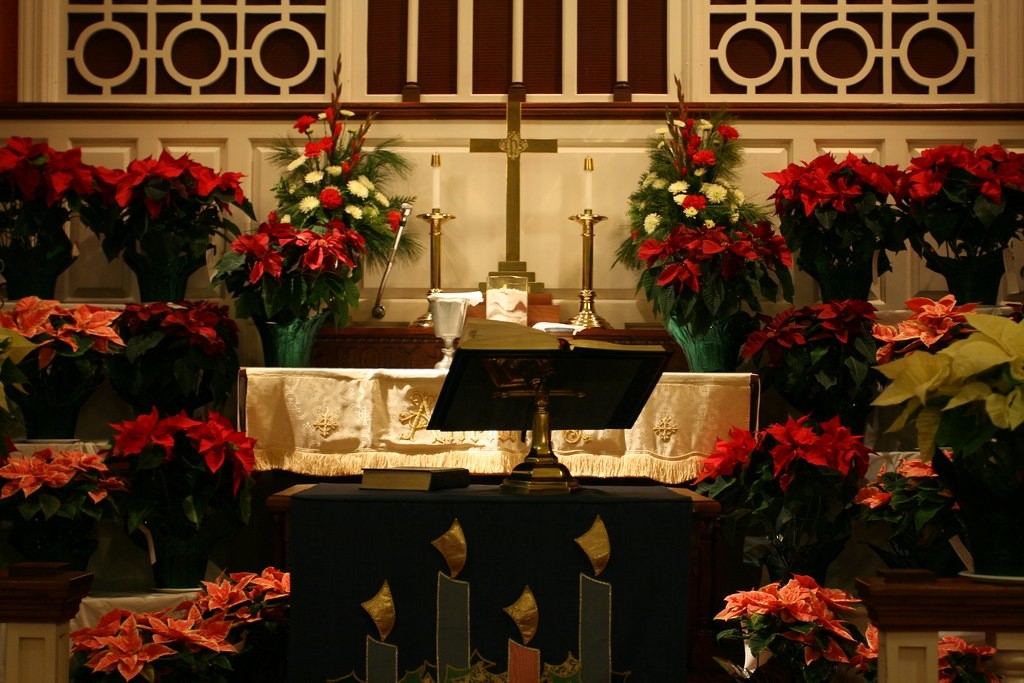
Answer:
[608,71,1023,683]
[1,54,425,683]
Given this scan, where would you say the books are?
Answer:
[359,466,471,491]
[426,317,672,433]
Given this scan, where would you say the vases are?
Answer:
[916,410,944,464]
[810,417,866,447]
[252,312,330,367]
[134,262,204,304]
[944,257,1003,307]
[124,519,238,587]
[6,529,98,570]
[662,317,767,378]
[812,268,871,302]
[7,255,77,301]
[125,392,195,420]
[762,545,840,587]
[20,405,81,439]
[857,539,942,584]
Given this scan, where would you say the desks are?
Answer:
[265,484,722,683]
[238,322,761,683]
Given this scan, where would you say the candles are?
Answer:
[583,157,593,214]
[430,152,441,214]
